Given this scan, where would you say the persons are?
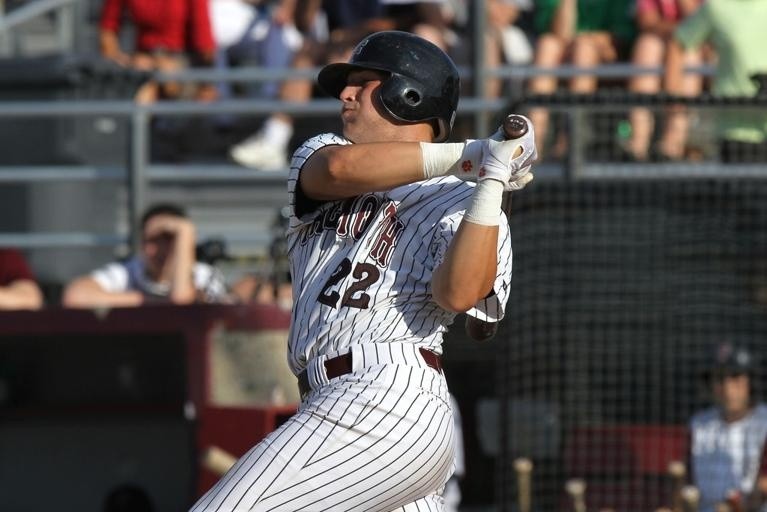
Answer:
[60,200,297,311]
[1,248,45,315]
[95,1,766,172]
[684,343,766,511]
[186,28,541,512]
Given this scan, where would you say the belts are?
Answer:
[298,346,442,401]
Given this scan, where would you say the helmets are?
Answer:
[701,340,753,387]
[317,30,461,142]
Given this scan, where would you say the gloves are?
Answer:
[457,115,538,190]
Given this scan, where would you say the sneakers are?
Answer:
[231,139,286,169]
